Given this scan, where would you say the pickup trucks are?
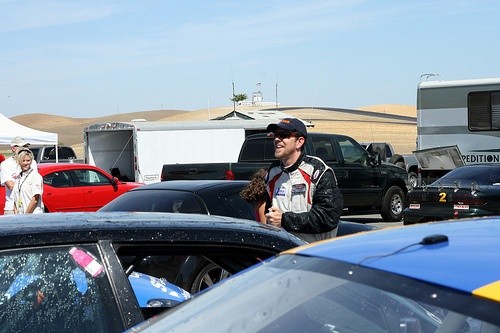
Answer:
[159,130,409,222]
[325,142,417,188]
[26,143,85,181]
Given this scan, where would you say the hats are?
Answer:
[10,137,30,147]
[266,118,307,136]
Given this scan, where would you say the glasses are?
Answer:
[272,131,299,139]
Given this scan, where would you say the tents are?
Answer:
[0,113,58,163]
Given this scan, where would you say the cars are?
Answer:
[95,177,258,223]
[0,154,147,216]
[0,211,310,333]
[1,256,193,308]
[124,215,500,333]
[403,163,500,224]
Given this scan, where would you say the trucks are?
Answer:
[83,116,283,185]
[417,73,500,189]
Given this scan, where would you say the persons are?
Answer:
[10,148,44,215]
[240,169,268,225]
[0,136,38,215]
[264,118,344,244]
